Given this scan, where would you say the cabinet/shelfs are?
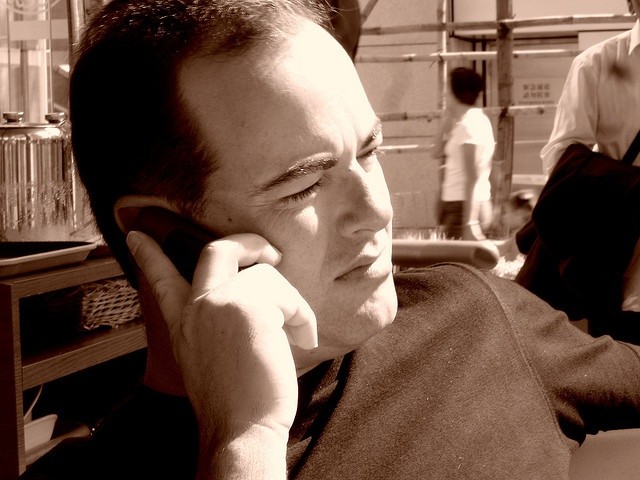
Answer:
[1,257,147,478]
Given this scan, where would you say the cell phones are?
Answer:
[129,209,257,287]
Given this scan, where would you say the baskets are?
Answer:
[76,280,141,330]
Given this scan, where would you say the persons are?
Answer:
[437,68,497,240]
[540,2,640,344]
[21,1,639,476]
[505,189,538,243]
